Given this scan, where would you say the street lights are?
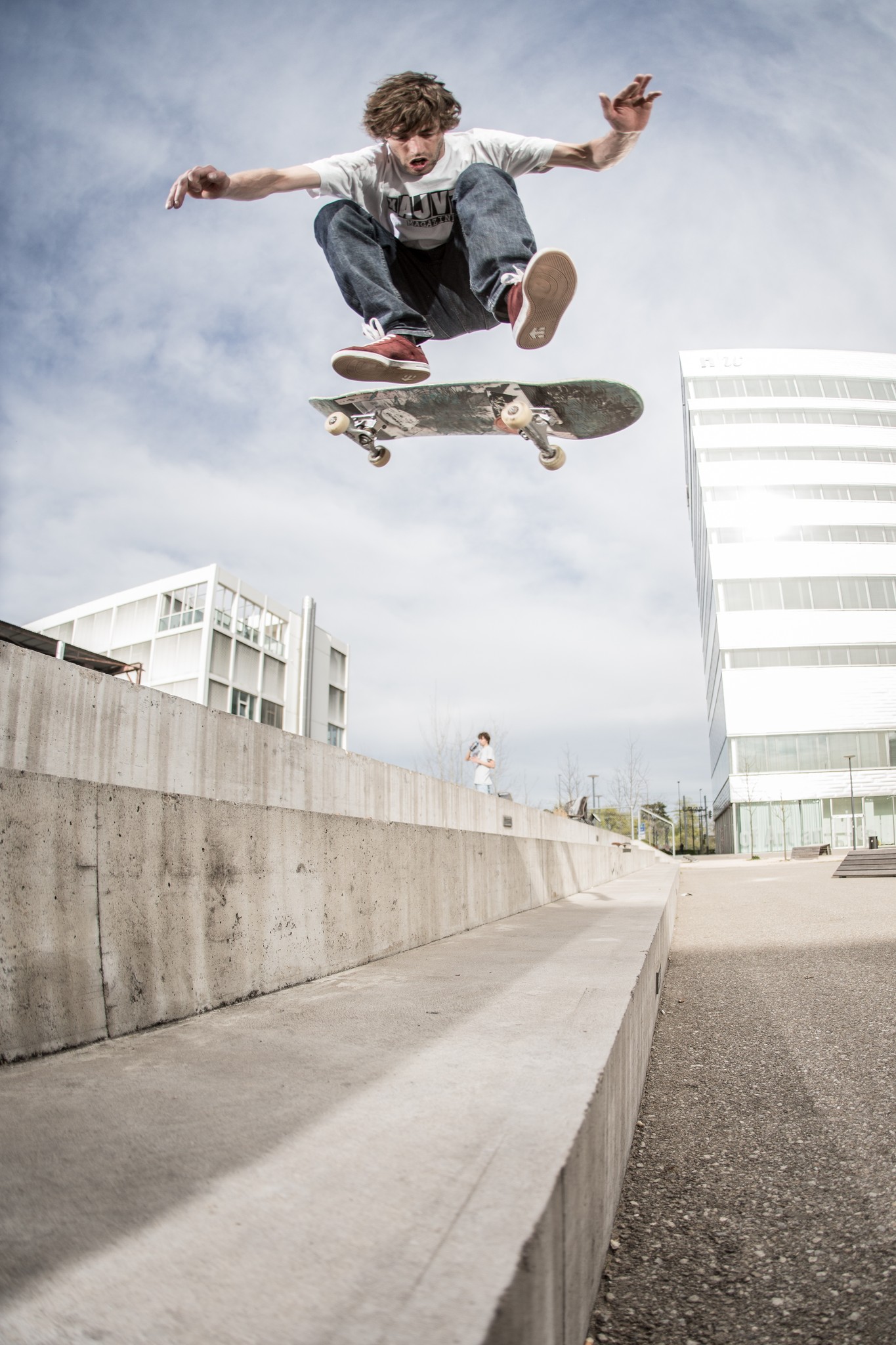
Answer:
[843,755,857,850]
[677,781,683,855]
[588,775,604,827]
[699,789,704,855]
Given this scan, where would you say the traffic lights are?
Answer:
[709,810,712,819]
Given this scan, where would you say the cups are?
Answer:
[470,741,479,751]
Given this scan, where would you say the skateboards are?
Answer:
[306,377,646,471]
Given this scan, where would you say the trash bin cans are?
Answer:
[869,836,878,849]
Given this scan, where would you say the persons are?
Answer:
[466,733,496,796]
[162,67,661,387]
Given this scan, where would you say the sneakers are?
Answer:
[501,248,578,350]
[329,318,432,384]
